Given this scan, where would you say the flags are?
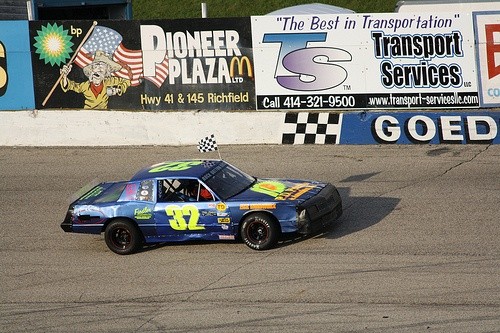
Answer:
[196,133,222,153]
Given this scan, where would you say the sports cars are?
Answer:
[59,158,343,252]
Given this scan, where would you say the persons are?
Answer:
[191,178,224,201]
